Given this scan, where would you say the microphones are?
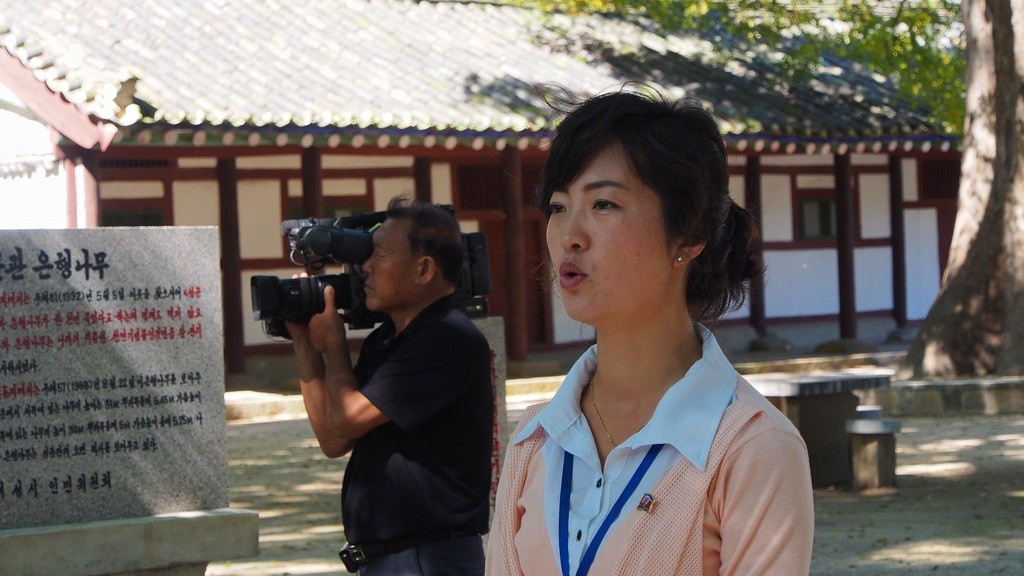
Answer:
[282,217,335,237]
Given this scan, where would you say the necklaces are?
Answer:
[590,381,615,447]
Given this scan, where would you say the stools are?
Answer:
[847,404,903,489]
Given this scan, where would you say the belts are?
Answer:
[347,526,480,564]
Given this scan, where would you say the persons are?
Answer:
[285,205,494,576]
[484,91,814,576]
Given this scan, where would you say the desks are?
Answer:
[739,368,896,489]
